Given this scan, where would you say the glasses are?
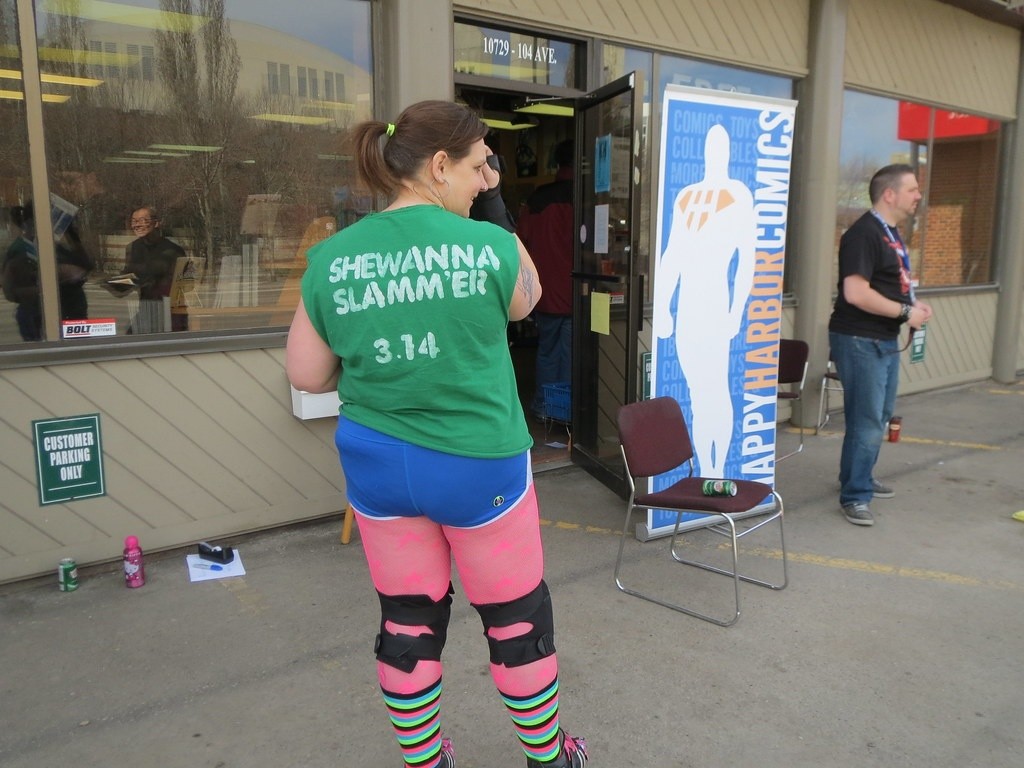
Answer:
[129,217,154,225]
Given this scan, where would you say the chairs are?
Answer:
[614,396,788,626]
[815,349,841,440]
[776,339,809,461]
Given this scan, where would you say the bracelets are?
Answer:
[897,302,911,324]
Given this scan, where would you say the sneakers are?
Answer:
[867,478,896,498]
[428,734,455,768]
[527,725,588,768]
[841,500,874,526]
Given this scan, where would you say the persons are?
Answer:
[0,201,97,342]
[286,101,588,768]
[828,164,932,526]
[104,207,194,334]
[515,161,576,422]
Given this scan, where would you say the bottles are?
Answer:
[122,536,145,587]
[888,416,901,442]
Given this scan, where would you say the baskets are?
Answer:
[541,380,572,422]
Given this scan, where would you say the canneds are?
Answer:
[702,480,737,497]
[58,558,80,592]
